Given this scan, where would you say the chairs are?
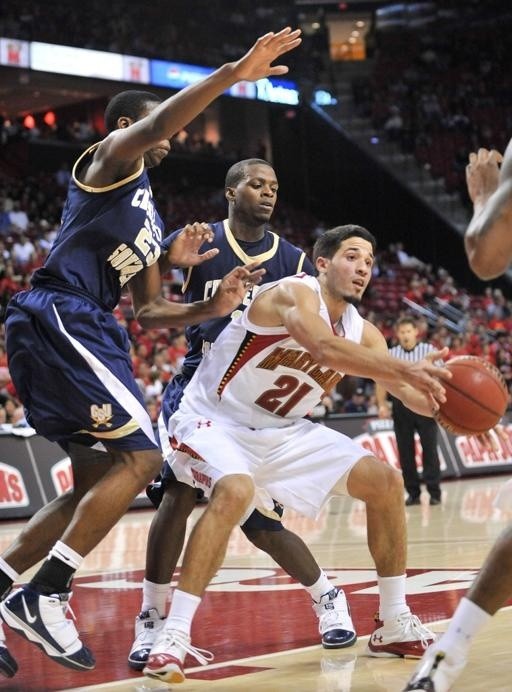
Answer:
[2,169,512,396]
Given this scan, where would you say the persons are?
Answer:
[128,158,356,672]
[1,26,303,677]
[0,61,511,429]
[402,141,512,691]
[389,318,444,505]
[142,224,453,682]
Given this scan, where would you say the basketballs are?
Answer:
[431,355,507,435]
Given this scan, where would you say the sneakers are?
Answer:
[368,606,468,692]
[312,588,357,649]
[128,608,214,683]
[405,496,441,505]
[0,584,97,678]
